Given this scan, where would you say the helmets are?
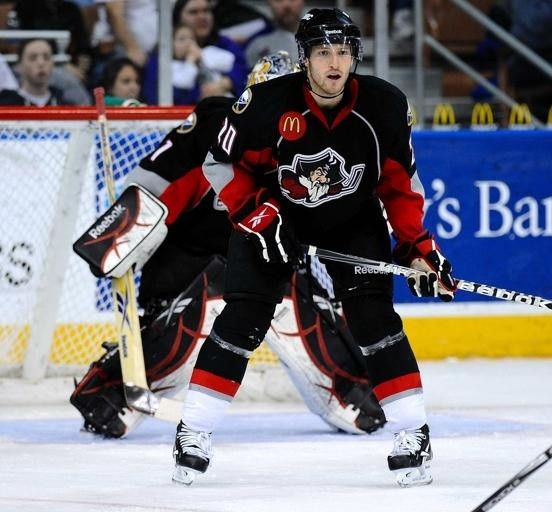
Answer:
[296,7,363,61]
[247,51,301,87]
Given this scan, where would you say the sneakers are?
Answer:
[175,420,211,472]
[388,424,430,470]
[90,382,129,437]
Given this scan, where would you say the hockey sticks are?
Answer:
[95,87,184,424]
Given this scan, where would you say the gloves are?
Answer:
[229,186,304,264]
[393,229,457,302]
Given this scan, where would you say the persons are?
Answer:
[1,0,306,109]
[69,47,388,438]
[165,5,457,473]
